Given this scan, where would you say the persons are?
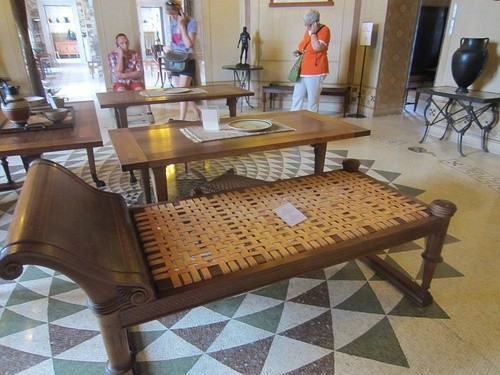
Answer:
[292,10,331,114]
[237,26,251,64]
[107,33,155,123]
[165,0,201,120]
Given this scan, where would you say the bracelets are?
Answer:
[310,31,317,36]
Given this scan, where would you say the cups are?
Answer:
[53,97,64,108]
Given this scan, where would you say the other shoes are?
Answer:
[147,112,154,124]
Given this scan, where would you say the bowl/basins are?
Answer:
[24,96,45,110]
[45,107,69,124]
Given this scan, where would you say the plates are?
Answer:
[226,119,273,132]
[163,87,190,94]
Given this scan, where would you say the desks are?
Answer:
[96,83,254,129]
[0,100,106,191]
[108,109,370,205]
[416,86,500,156]
[220,65,263,112]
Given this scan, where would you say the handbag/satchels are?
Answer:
[164,52,189,72]
[287,54,303,81]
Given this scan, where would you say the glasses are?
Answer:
[166,2,175,8]
[305,23,312,26]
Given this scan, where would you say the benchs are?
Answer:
[262,83,351,117]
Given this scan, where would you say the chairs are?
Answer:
[0,158,457,375]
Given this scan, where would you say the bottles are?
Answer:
[0,82,30,127]
[451,36,490,94]
